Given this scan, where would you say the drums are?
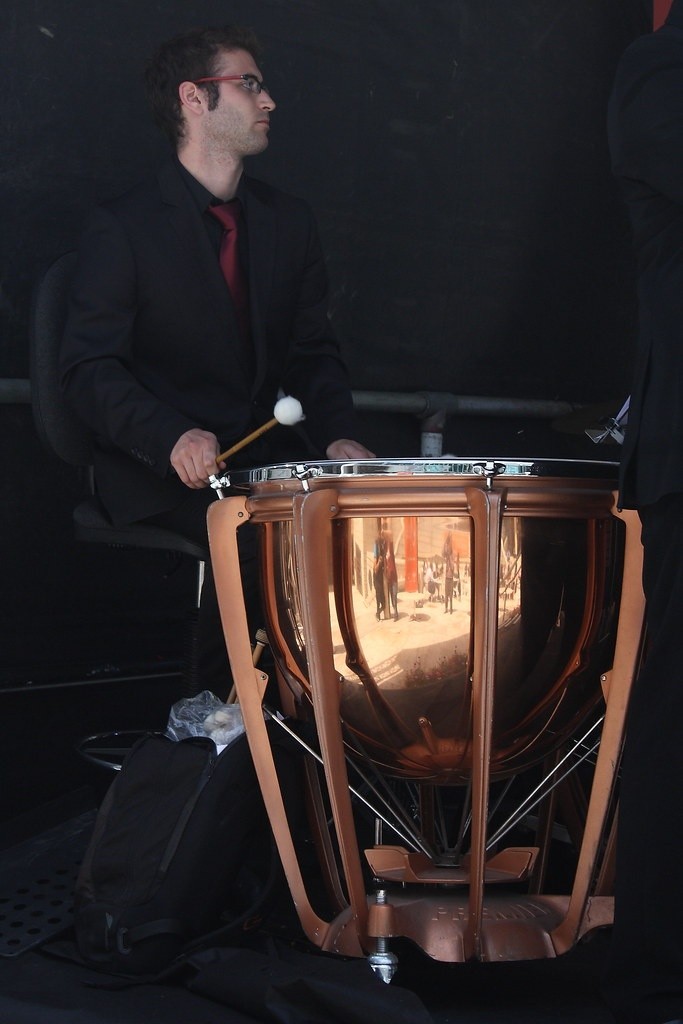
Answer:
[203,455,651,964]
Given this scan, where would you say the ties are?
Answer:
[208,202,249,336]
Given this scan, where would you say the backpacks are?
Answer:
[80,700,273,972]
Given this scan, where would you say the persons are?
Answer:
[58,27,379,712]
[370,516,513,622]
[615,0,683,1023]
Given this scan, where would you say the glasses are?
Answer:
[194,74,262,94]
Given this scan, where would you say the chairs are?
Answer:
[30,245,352,772]
